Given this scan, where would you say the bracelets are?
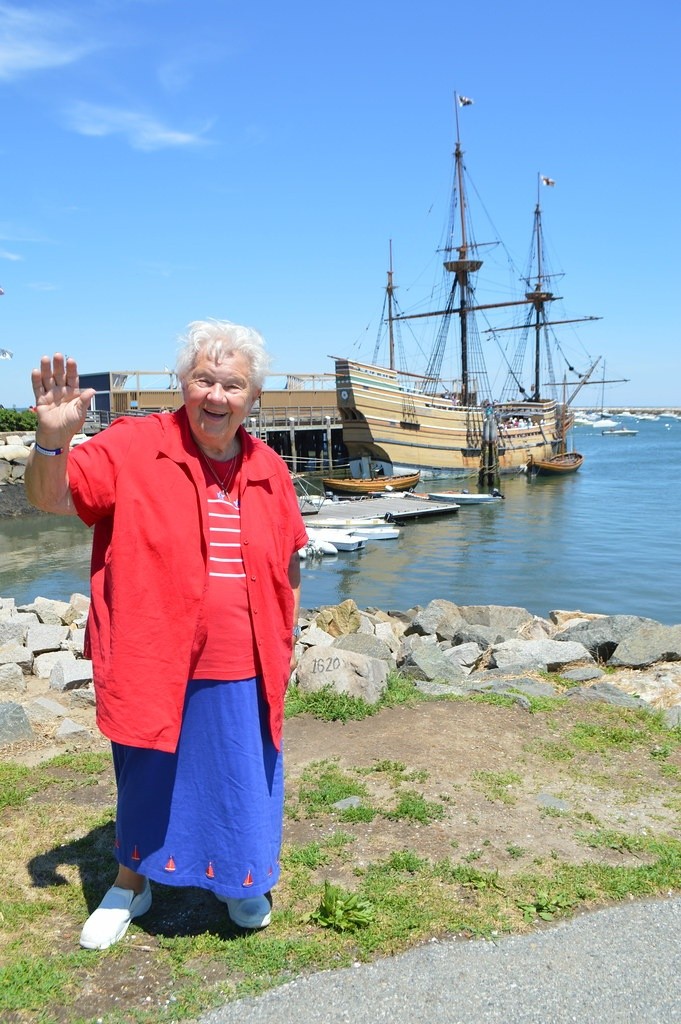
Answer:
[33,442,69,458]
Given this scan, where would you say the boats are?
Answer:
[525,451,584,476]
[322,470,421,498]
[297,517,400,559]
[368,489,503,504]
[602,425,639,437]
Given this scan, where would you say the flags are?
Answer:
[541,177,555,186]
[457,96,473,107]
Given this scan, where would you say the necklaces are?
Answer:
[193,433,238,502]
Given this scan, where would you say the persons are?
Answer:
[497,417,546,428]
[24,316,309,952]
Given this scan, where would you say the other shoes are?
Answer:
[215,892,271,928]
[80,875,152,949]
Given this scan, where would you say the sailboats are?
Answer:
[322,90,630,484]
